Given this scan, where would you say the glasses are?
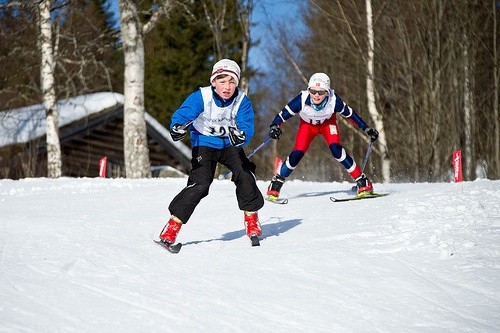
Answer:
[308,88,328,95]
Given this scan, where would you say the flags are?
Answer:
[99,157,106,178]
[452,150,462,183]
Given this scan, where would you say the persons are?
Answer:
[161,59,264,246]
[266,72,380,200]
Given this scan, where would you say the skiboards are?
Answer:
[265,192,390,204]
[153,232,261,254]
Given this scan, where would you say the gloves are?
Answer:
[268,125,282,140]
[228,129,246,149]
[170,123,187,142]
[365,128,379,142]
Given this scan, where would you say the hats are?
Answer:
[210,58,240,88]
[308,72,330,93]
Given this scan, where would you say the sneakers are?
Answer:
[243,212,261,236]
[160,215,182,244]
[267,175,285,200]
[356,178,374,196]
